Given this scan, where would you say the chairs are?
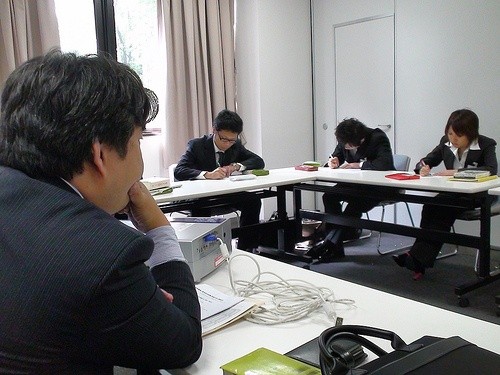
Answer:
[170,155,500,274]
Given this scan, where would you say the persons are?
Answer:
[390,110,498,276]
[172,111,265,255]
[303,118,400,262]
[0,55,203,375]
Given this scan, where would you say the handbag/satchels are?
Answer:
[318,325,500,375]
[259,211,294,246]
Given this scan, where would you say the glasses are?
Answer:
[217,131,238,143]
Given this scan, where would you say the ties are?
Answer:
[216,152,224,167]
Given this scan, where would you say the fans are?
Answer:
[142,88,160,137]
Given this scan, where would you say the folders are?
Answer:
[447,175,498,183]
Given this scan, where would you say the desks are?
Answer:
[278,166,500,308]
[118,170,316,264]
[157,247,500,375]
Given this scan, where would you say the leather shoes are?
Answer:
[307,240,335,256]
[315,227,329,237]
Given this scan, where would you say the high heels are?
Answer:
[392,253,414,267]
[406,256,424,281]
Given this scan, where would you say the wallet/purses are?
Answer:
[284,336,368,369]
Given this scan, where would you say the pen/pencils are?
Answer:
[421,160,432,177]
[217,162,223,168]
[329,153,339,168]
